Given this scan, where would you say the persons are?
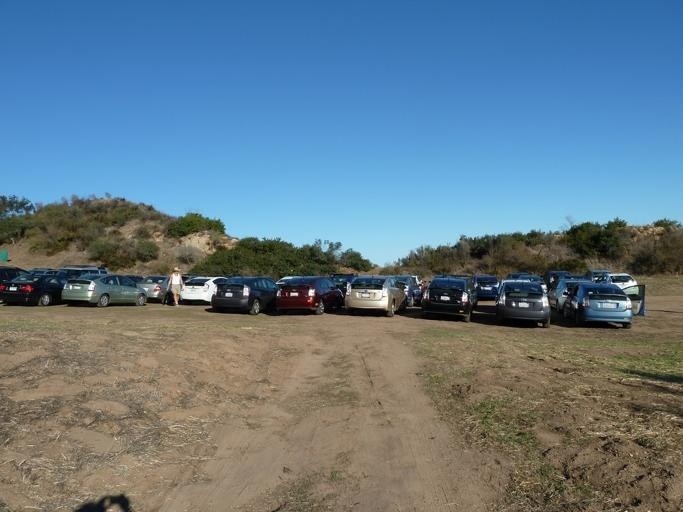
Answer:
[165,267,186,306]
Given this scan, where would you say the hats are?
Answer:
[172,268,181,272]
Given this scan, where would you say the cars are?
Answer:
[562,282,634,329]
[215,276,275,315]
[281,266,647,309]
[135,276,170,304]
[60,273,148,307]
[420,277,477,323]
[179,276,228,305]
[1,274,64,306]
[344,275,408,317]
[0,264,107,302]
[495,281,553,328]
[274,275,343,315]
